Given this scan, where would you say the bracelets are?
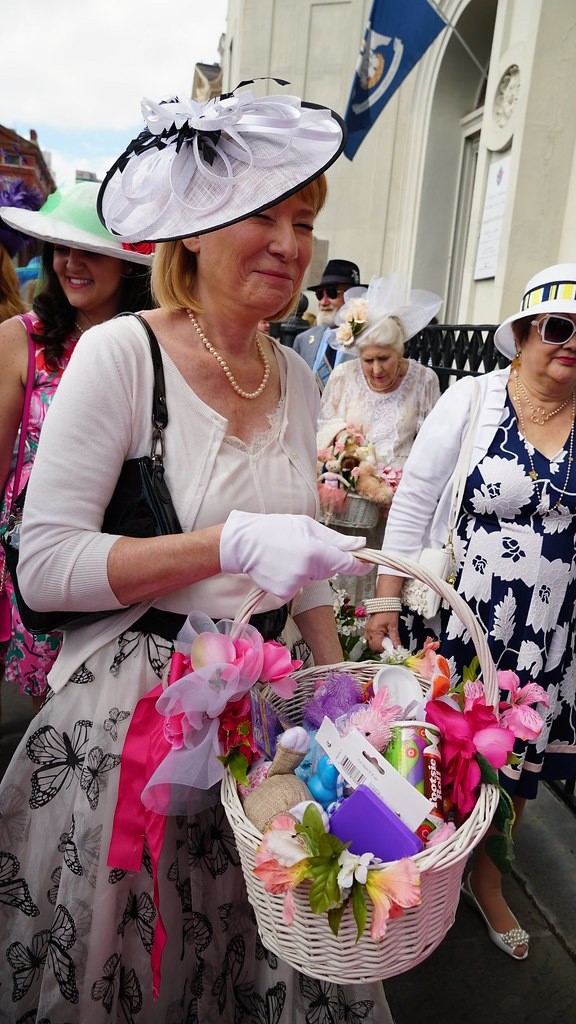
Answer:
[362,597,402,613]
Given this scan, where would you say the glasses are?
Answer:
[315,288,347,301]
[528,315,576,345]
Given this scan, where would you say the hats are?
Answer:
[308,259,376,299]
[0,179,157,265]
[97,77,347,244]
[494,264,576,361]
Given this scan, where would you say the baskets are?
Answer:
[222,549,498,985]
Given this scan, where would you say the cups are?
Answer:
[359,665,423,708]
[385,718,445,848]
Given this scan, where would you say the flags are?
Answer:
[343,0,448,162]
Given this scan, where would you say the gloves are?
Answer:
[220,510,377,600]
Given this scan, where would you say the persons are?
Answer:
[0,178,156,716]
[291,259,369,386]
[364,263,576,959]
[316,275,442,609]
[0,76,394,1024]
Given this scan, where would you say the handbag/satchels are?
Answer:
[3,312,184,633]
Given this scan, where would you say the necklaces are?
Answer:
[75,322,84,333]
[514,372,576,512]
[185,306,271,399]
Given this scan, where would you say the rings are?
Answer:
[363,638,368,645]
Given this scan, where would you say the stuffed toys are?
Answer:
[237,683,462,855]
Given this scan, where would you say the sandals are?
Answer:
[460,871,528,959]
